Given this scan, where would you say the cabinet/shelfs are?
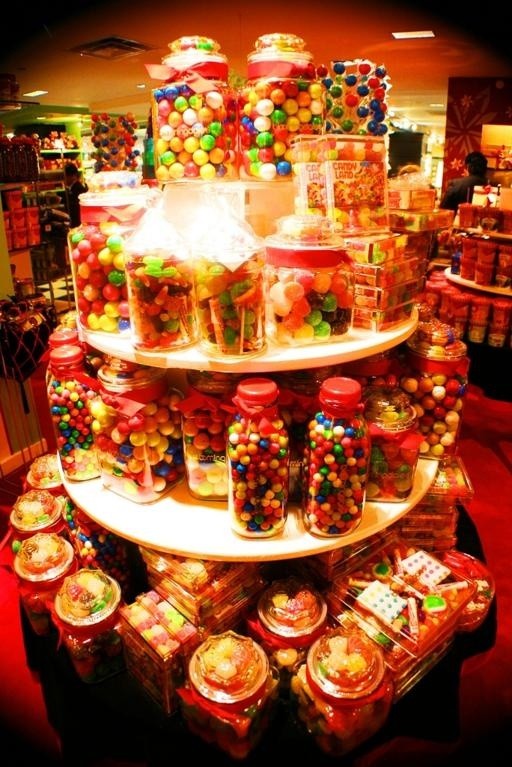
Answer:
[0,98,511,766]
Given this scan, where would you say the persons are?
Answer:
[437,150,491,220]
[48,160,86,230]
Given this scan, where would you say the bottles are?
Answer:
[2,37,512,767]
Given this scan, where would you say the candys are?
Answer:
[12,453,496,760]
[66,33,427,359]
[44,309,469,540]
